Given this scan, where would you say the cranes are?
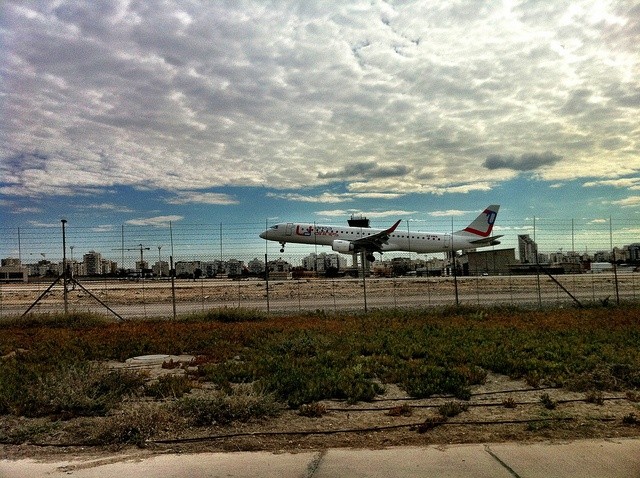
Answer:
[111,243,150,267]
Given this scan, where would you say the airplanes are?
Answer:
[258,204,505,262]
